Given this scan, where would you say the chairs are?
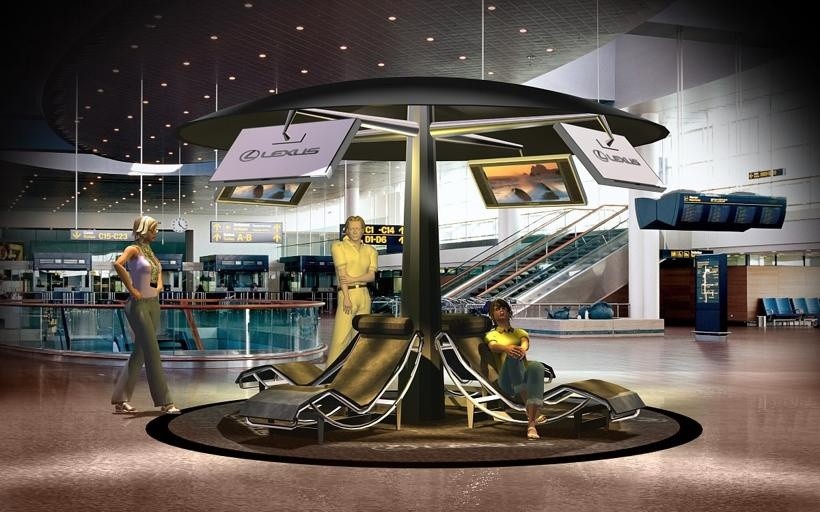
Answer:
[434,314,647,434]
[236,311,393,436]
[759,297,820,328]
[233,315,425,444]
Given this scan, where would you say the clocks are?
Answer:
[171,217,188,233]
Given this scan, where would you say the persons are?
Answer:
[324,214,380,371]
[109,215,184,417]
[44,308,59,336]
[486,299,549,440]
